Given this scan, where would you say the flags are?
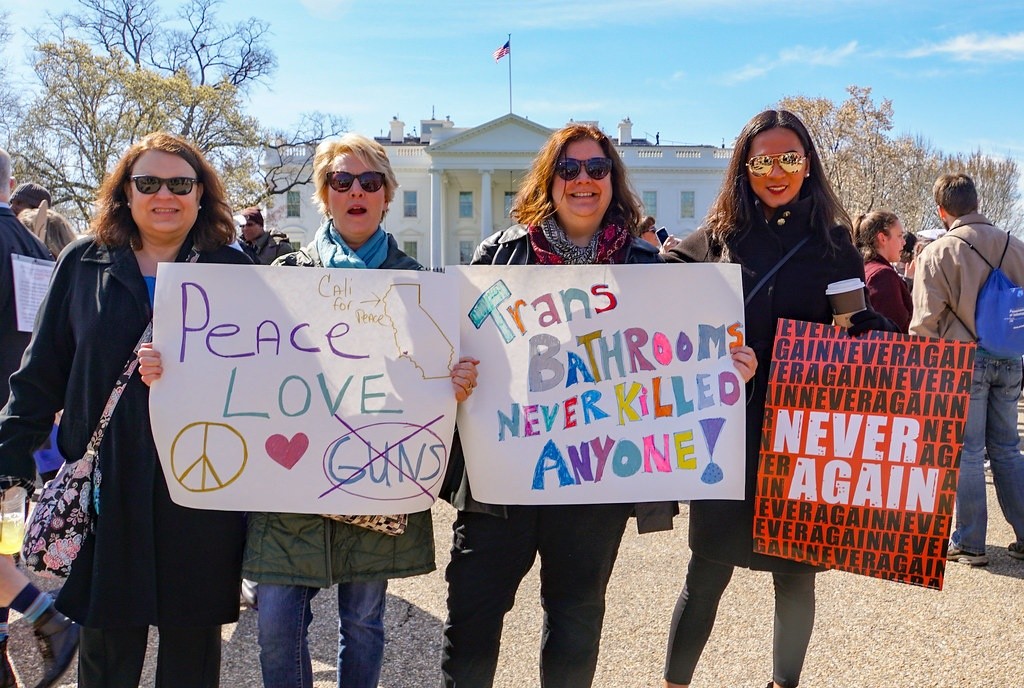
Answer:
[492,40,510,64]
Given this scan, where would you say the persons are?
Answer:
[633,216,677,254]
[439,125,758,688]
[0,148,80,688]
[855,211,948,337]
[659,110,902,688]
[240,207,277,265]
[0,132,250,688]
[137,131,481,688]
[907,173,1024,564]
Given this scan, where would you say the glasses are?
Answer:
[643,226,656,234]
[128,175,198,195]
[239,222,255,227]
[325,170,387,193]
[555,157,613,181]
[744,152,807,178]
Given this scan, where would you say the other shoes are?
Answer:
[945,539,988,565]
[1007,541,1024,559]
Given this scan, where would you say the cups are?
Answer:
[825,277,866,327]
[0,487,28,555]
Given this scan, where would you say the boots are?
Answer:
[31,602,81,688]
[0,635,17,687]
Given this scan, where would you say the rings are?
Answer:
[466,385,472,391]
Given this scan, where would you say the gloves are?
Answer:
[846,310,894,338]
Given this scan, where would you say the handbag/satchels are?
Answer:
[20,450,101,579]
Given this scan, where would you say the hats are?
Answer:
[237,206,264,227]
[9,183,51,209]
[917,228,947,240]
[639,215,655,231]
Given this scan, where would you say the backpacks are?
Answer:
[944,231,1024,357]
